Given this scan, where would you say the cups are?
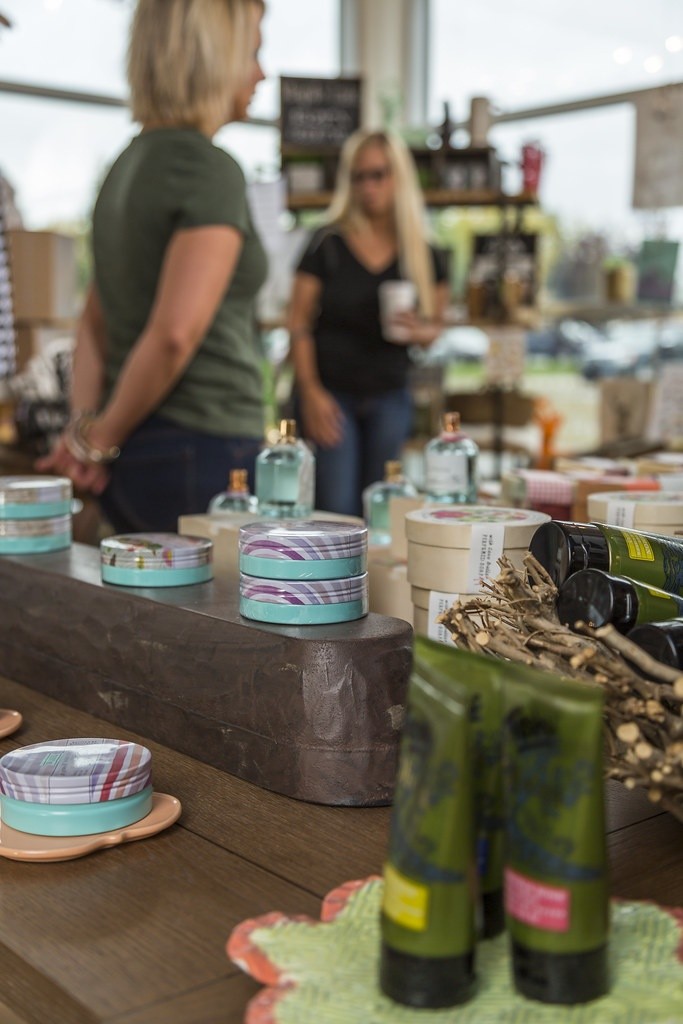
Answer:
[381,282,416,343]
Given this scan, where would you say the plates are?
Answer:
[0,794,181,862]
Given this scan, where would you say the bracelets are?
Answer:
[58,419,124,463]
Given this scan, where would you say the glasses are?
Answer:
[352,169,385,182]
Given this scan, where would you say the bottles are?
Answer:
[423,412,480,505]
[253,419,317,517]
[363,461,418,548]
[207,469,257,517]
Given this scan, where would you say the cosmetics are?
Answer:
[1,415,683,1010]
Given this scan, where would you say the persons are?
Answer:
[29,0,271,540]
[282,126,443,525]
